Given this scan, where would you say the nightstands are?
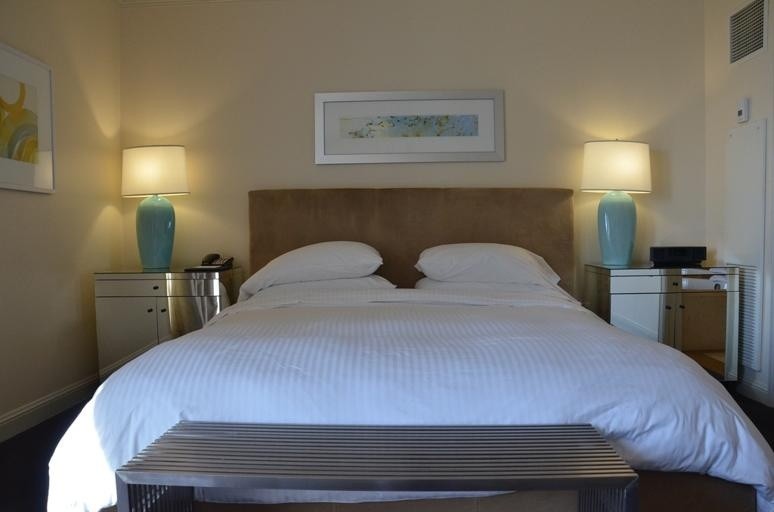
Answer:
[91,265,246,382]
[581,260,741,389]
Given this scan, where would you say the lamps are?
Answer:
[117,143,191,272]
[579,136,654,269]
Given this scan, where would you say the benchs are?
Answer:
[113,421,640,510]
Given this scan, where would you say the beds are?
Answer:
[106,185,762,510]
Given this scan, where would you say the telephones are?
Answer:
[201,254,233,270]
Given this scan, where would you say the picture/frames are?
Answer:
[0,41,58,197]
[314,88,506,166]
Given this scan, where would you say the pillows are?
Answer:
[415,242,560,287]
[237,238,381,302]
[415,278,575,304]
[250,274,397,297]
